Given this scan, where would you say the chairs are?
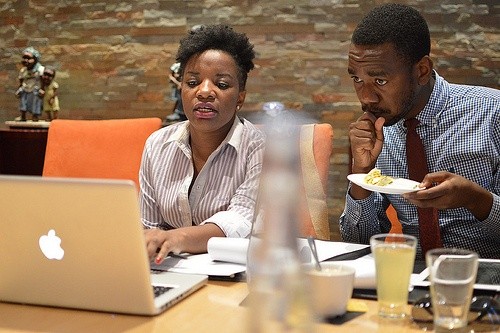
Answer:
[255,122,332,242]
[42,118,162,192]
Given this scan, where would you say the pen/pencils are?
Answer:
[155,247,189,261]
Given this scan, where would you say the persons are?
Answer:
[14,47,60,122]
[138,25,269,264]
[166,25,202,122]
[340,3,500,259]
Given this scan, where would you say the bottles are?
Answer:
[247,112,320,333]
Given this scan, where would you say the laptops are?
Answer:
[0,175,209,315]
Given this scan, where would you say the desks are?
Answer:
[1,276,500,333]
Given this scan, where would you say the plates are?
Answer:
[347,173,426,195]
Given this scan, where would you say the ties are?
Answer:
[402,117,444,260]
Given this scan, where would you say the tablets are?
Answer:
[410,258,500,292]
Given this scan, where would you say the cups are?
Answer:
[369,234,417,320]
[425,248,478,333]
[301,263,356,321]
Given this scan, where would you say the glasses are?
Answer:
[411,296,495,326]
